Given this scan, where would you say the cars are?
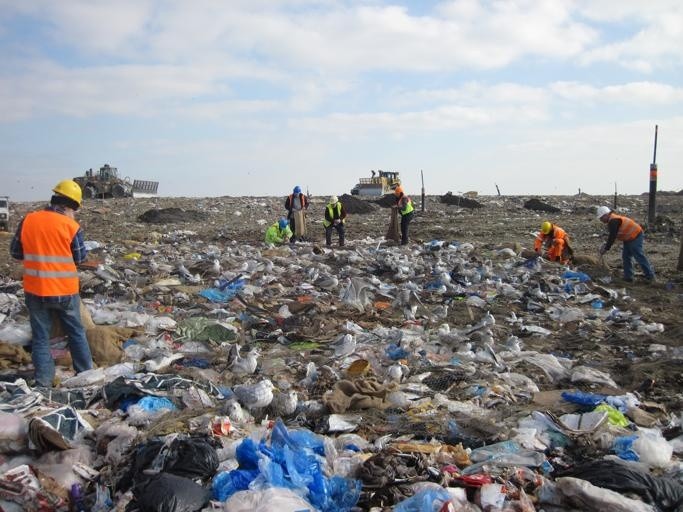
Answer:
[350,184,361,195]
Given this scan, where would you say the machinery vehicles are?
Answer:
[358,170,401,197]
[71,164,159,199]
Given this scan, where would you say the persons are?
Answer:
[321,195,348,247]
[265,218,293,247]
[9,179,96,388]
[284,185,309,243]
[596,206,657,284]
[390,186,416,245]
[534,221,575,267]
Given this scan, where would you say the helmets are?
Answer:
[293,186,301,194]
[279,218,287,230]
[394,187,402,194]
[595,205,611,219]
[540,222,551,234]
[52,180,83,209]
[328,196,338,204]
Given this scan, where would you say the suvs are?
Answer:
[0,196,10,232]
